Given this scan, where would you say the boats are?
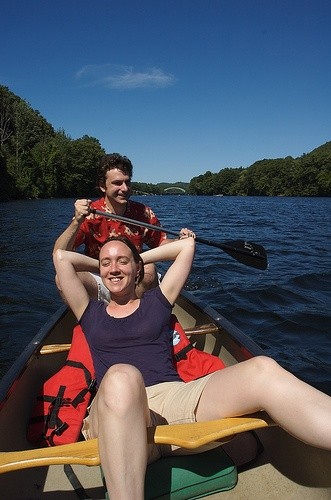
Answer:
[0,249,331,500]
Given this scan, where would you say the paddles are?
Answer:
[90,208,268,271]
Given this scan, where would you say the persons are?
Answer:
[52,236,331,500]
[52,151,196,310]
